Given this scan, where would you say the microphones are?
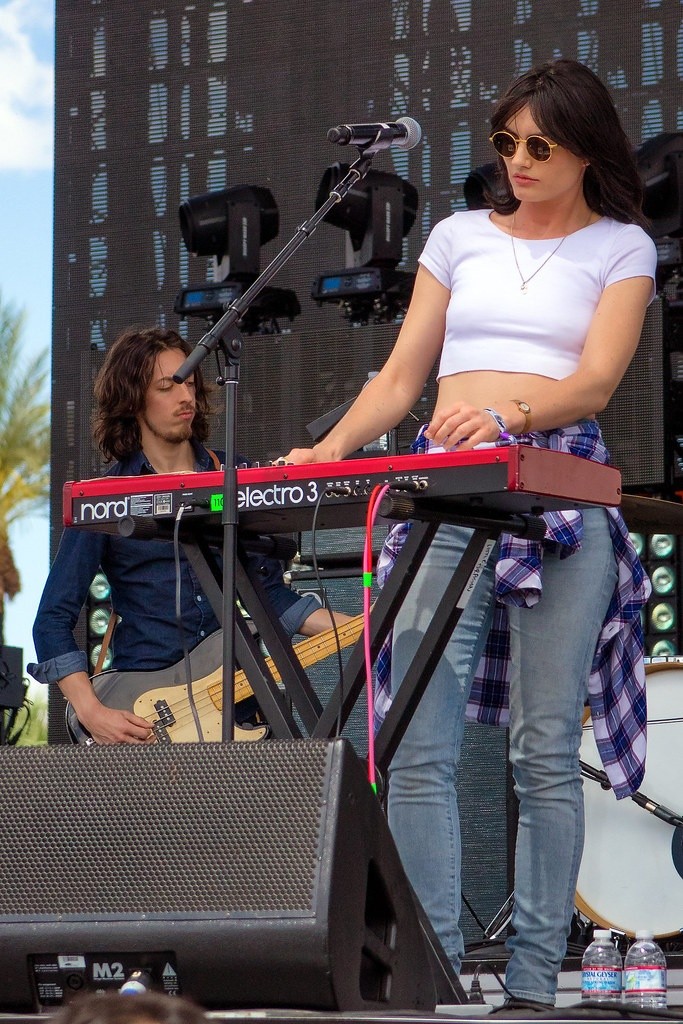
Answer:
[327,117,421,149]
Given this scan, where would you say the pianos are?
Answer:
[61,442,625,1007]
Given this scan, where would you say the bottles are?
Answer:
[361,371,388,451]
[624,930,667,1010]
[581,930,623,1004]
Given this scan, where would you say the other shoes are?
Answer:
[492,998,554,1015]
[119,969,153,996]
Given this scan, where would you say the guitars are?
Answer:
[64,598,375,742]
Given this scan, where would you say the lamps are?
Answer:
[638,131,683,295]
[173,185,301,336]
[312,162,418,326]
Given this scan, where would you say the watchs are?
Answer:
[510,399,532,433]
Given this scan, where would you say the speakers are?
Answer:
[290,568,524,975]
[0,743,437,1017]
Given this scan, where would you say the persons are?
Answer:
[32,326,356,1000]
[286,58,656,1019]
[45,989,211,1024]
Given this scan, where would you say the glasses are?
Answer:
[489,130,561,162]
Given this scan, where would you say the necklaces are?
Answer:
[512,208,594,294]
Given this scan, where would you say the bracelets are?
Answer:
[485,408,507,433]
